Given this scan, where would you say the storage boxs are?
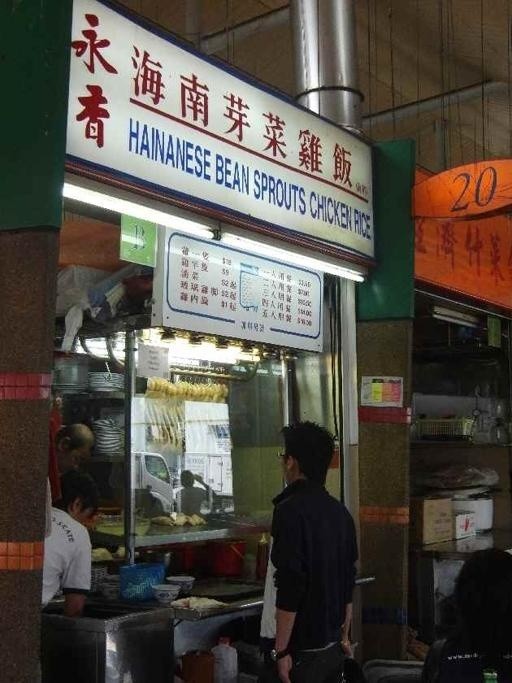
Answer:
[410,495,477,547]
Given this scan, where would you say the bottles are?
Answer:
[239,553,257,581]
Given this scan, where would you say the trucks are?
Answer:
[131,451,174,514]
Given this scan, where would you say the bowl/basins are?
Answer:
[100,581,122,600]
[167,574,195,595]
[152,582,181,606]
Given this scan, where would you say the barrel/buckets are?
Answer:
[207,539,246,576]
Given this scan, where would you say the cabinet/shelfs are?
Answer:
[66,391,138,466]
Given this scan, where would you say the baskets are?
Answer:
[119,562,166,601]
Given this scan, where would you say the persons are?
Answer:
[260,421,359,683]
[176,470,217,515]
[41,423,99,616]
[421,547,512,683]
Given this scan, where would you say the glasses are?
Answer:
[276,452,290,457]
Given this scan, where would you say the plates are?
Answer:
[87,370,125,391]
[52,383,88,395]
[91,418,125,456]
